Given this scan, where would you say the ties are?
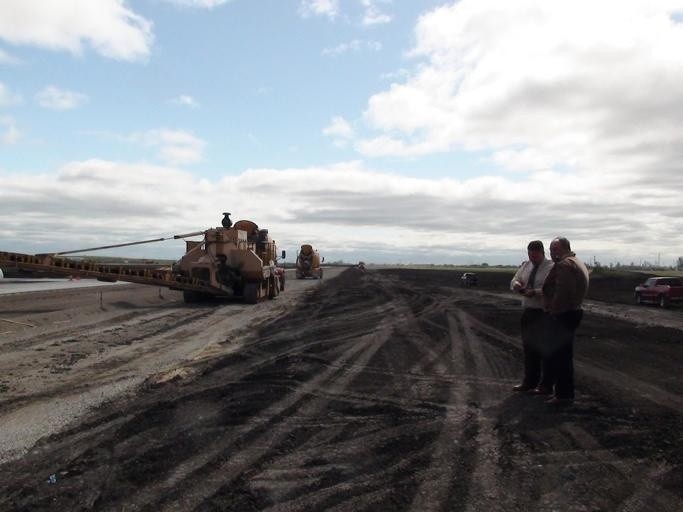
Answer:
[524,263,540,292]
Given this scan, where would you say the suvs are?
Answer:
[634,276,681,309]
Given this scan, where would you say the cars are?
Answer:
[459,272,480,287]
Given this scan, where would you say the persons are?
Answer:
[510,240,555,395]
[541,236,589,405]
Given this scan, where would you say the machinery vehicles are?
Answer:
[0,207,285,307]
[295,244,325,280]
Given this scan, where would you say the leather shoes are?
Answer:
[510,383,553,396]
[540,395,574,408]
